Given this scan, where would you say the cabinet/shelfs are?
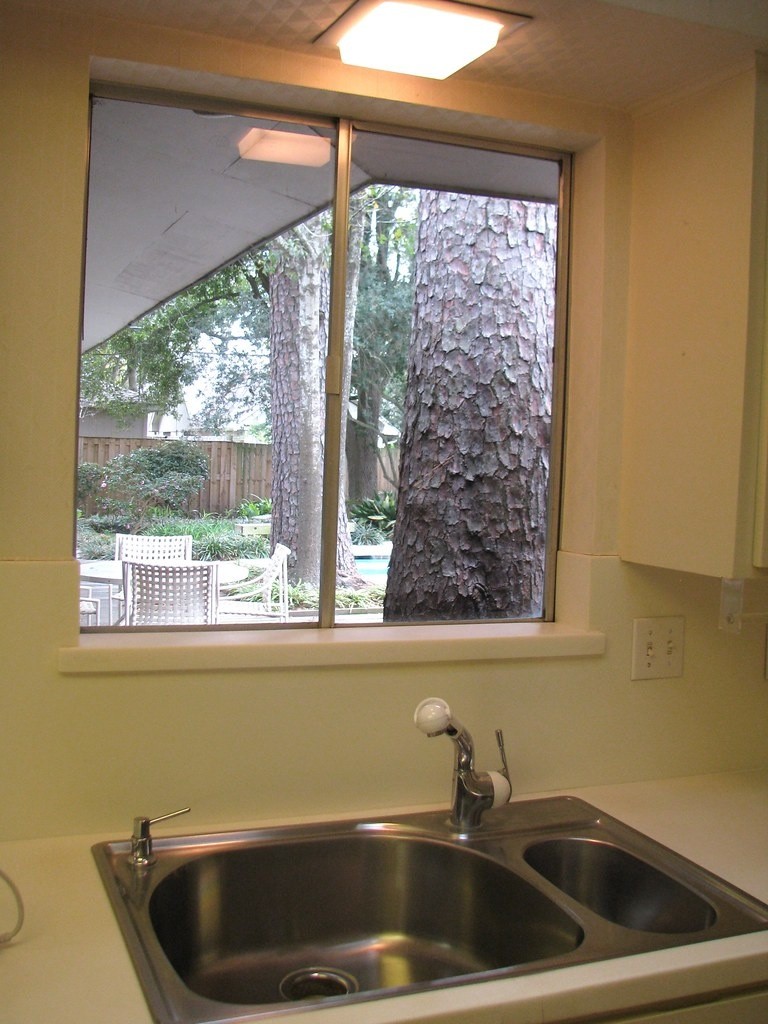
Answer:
[619,59,768,591]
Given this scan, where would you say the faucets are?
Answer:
[412,697,513,830]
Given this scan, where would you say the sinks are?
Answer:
[123,833,586,1024]
[518,828,768,965]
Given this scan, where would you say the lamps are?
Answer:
[312,0,531,87]
[237,126,333,168]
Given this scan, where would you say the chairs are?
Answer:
[113,533,193,618]
[80,584,113,626]
[220,544,291,624]
[119,560,218,626]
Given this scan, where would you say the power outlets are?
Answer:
[633,616,690,684]
[718,578,744,634]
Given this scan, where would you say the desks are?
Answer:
[79,560,250,626]
